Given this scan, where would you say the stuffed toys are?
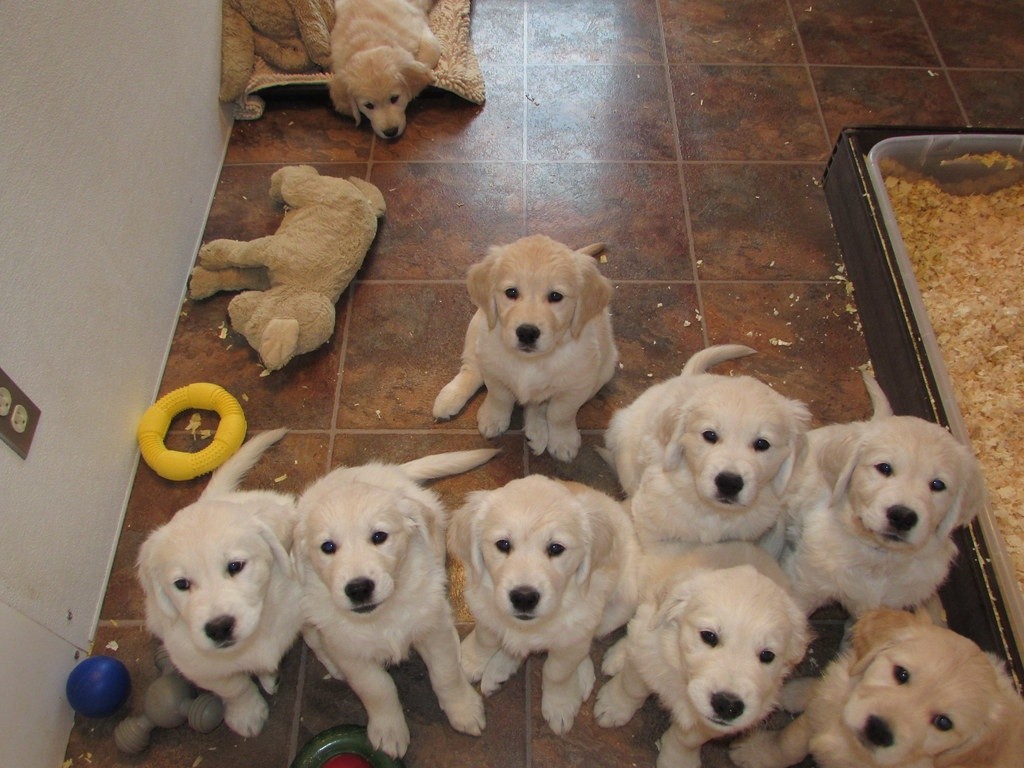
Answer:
[219,0,335,102]
[188,164,390,370]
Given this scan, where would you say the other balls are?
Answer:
[66,655,132,717]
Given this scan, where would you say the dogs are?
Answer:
[431,233,621,464]
[445,474,638,737]
[289,448,507,760]
[780,370,988,620]
[728,608,1024,768]
[133,425,300,738]
[592,540,819,768]
[328,0,443,140]
[593,342,813,557]
